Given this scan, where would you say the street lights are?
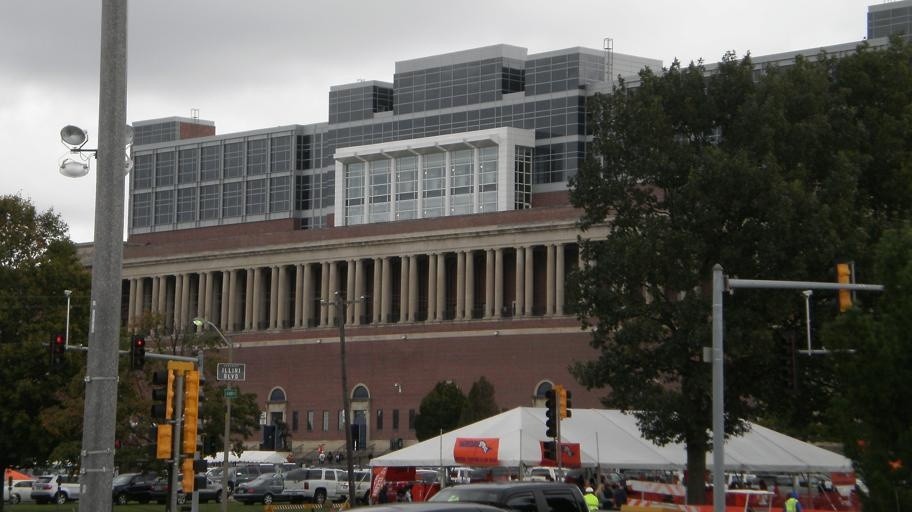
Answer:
[191,315,235,511]
[56,122,138,176]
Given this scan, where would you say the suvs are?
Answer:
[112,471,156,504]
[428,481,589,512]
[30,473,81,504]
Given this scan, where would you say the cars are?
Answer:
[4,479,36,504]
[339,501,507,512]
[164,461,371,505]
[148,474,233,506]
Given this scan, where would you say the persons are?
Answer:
[727,473,840,512]
[544,474,554,484]
[286,443,347,468]
[575,468,630,512]
[638,470,714,491]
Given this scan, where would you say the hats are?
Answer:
[586,487,593,492]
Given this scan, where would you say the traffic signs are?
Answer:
[215,361,246,382]
[224,388,240,398]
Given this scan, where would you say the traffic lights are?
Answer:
[183,368,206,455]
[180,458,209,493]
[560,386,572,419]
[48,331,66,372]
[116,438,121,448]
[152,367,174,421]
[130,333,147,370]
[545,388,557,439]
